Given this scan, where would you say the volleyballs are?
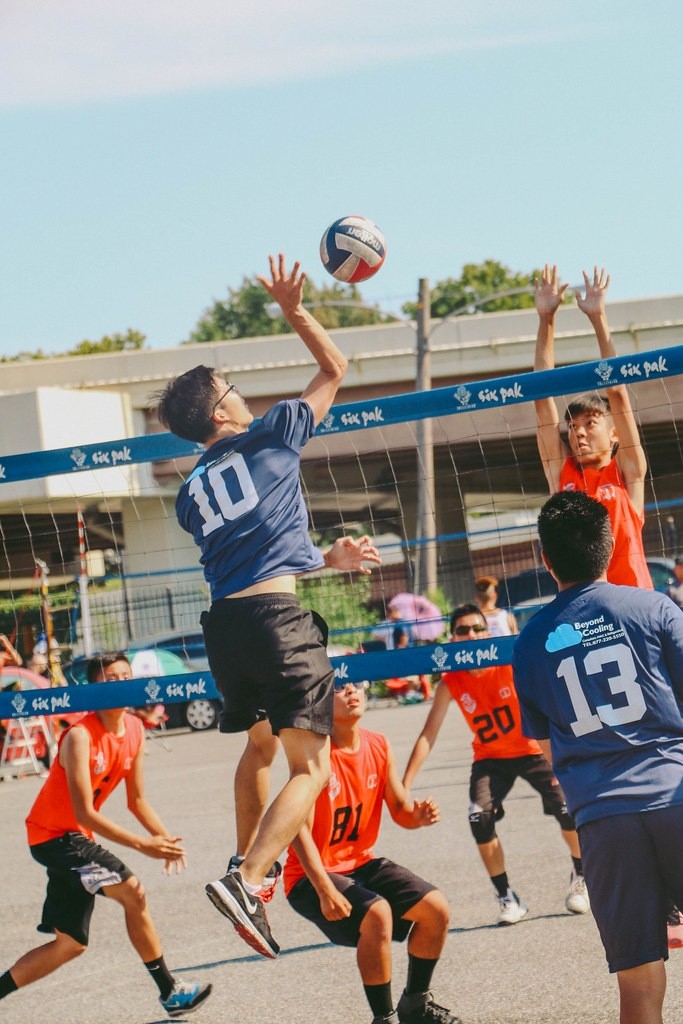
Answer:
[319,214,389,284]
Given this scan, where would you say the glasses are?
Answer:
[454,624,486,636]
[212,381,239,415]
[335,681,363,693]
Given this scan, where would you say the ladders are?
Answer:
[0,715,58,779]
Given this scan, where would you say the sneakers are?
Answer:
[394,988,462,1024]
[565,865,590,913]
[205,872,280,959]
[227,856,282,891]
[666,904,683,948]
[495,887,528,925]
[158,979,213,1017]
[371,1010,401,1024]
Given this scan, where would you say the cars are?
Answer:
[512,595,555,633]
[41,649,223,735]
[120,630,214,671]
[0,666,83,771]
[493,554,681,613]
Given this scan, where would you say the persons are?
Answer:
[474,575,520,638]
[665,555,683,610]
[510,488,683,1024]
[403,603,591,927]
[156,252,382,961]
[531,261,654,590]
[391,625,425,705]
[283,679,466,1024]
[0,649,213,1018]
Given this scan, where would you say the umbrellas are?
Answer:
[389,592,446,648]
[0,665,51,736]
[126,646,189,730]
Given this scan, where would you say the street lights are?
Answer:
[261,284,585,596]
